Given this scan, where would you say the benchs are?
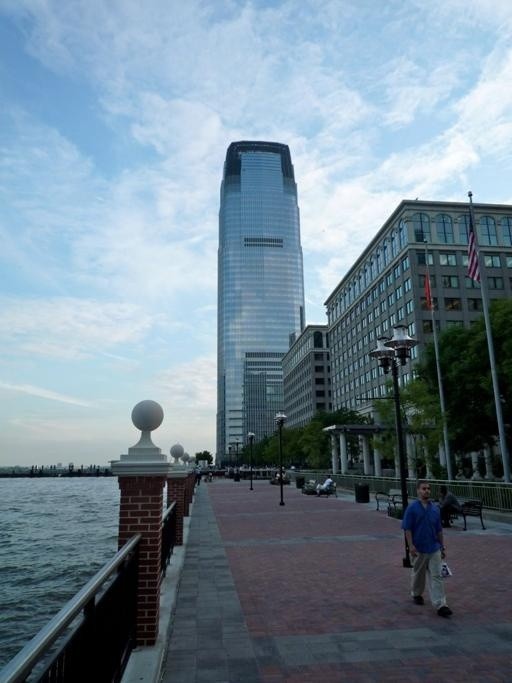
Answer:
[375,488,485,529]
[302,480,337,498]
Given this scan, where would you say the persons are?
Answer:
[275,469,286,480]
[454,467,484,481]
[314,474,333,497]
[437,484,462,528]
[193,462,215,487]
[400,479,452,616]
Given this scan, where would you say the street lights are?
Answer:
[370,325,419,568]
[234,437,241,481]
[274,413,288,505]
[247,432,256,489]
[228,447,232,478]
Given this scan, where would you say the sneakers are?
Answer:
[436,605,453,616]
[411,594,425,605]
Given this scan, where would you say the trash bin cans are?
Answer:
[354,482,369,503]
[234,474,240,481]
[296,476,305,488]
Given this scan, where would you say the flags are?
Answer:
[465,213,481,284]
[425,266,434,310]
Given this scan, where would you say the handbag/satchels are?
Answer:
[404,545,414,568]
[439,558,453,578]
